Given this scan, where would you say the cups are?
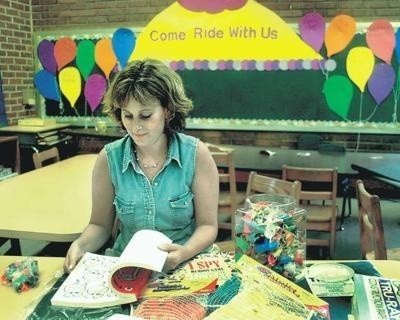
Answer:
[95,122,107,133]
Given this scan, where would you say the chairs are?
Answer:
[282,163,338,259]
[297,133,352,232]
[355,179,387,261]
[245,171,301,242]
[32,147,60,168]
[206,143,245,241]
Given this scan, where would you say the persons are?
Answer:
[64,59,220,276]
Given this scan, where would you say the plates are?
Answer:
[310,264,356,282]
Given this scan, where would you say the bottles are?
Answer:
[233,193,307,283]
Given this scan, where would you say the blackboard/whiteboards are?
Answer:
[33,22,400,134]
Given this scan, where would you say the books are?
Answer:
[50,229,173,309]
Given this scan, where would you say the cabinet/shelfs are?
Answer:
[0,136,20,178]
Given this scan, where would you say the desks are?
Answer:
[211,143,358,189]
[351,152,400,192]
[0,122,72,174]
[0,256,400,320]
[0,154,97,243]
[60,127,128,149]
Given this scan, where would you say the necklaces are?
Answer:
[133,147,166,169]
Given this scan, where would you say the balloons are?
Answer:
[299,12,400,152]
[32,27,136,117]
